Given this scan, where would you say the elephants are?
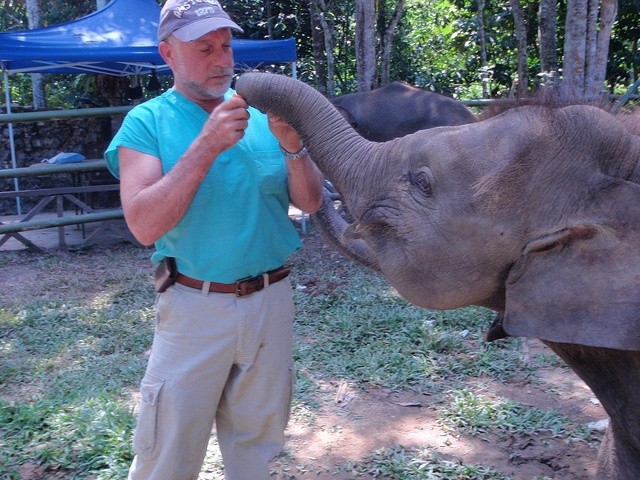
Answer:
[234,71,639,480]
[328,80,479,142]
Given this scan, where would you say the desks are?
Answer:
[29,159,107,238]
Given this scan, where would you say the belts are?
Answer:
[173,266,292,297]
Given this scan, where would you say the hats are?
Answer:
[156,0,244,44]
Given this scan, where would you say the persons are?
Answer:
[102,0,325,479]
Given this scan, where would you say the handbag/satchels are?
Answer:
[41,152,85,164]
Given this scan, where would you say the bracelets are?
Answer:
[278,141,307,160]
